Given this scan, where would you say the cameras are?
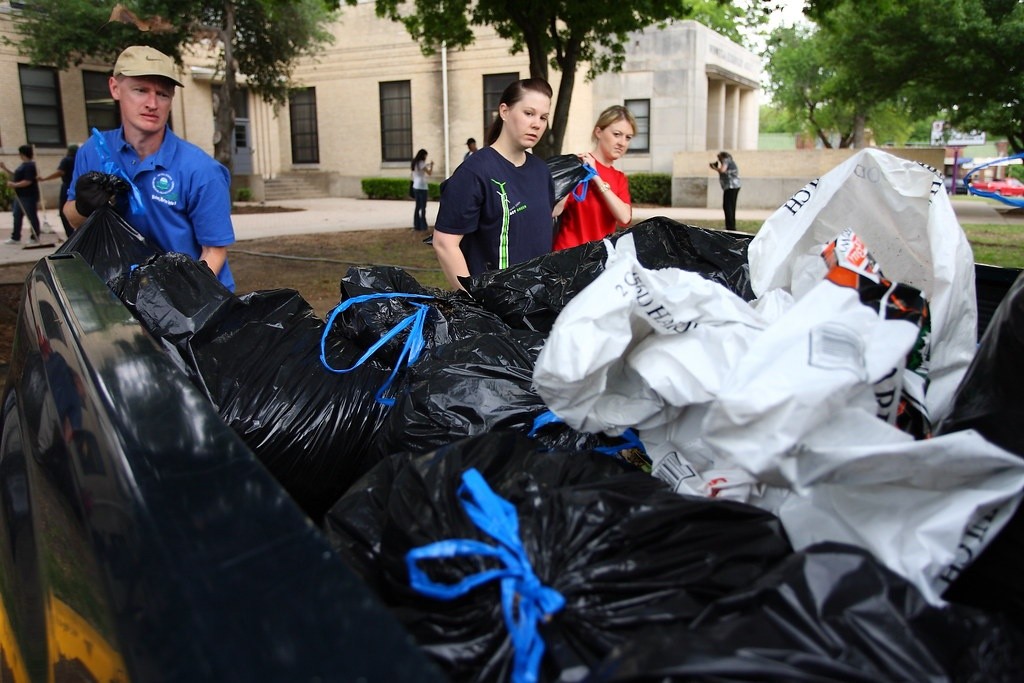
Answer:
[710,161,718,168]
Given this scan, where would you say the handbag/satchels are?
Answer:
[409,180,415,199]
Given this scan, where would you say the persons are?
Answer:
[62,46,237,294]
[710,152,741,231]
[552,105,637,252]
[0,145,40,244]
[464,138,479,159]
[432,79,555,298]
[410,149,433,231]
[36,144,79,237]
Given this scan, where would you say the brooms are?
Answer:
[13,188,56,250]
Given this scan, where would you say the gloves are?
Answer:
[75,173,110,218]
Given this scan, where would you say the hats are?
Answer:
[465,138,475,145]
[113,45,185,88]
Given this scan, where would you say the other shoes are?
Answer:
[4,238,21,244]
[25,238,36,244]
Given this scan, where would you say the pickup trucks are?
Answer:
[1,218,1023,683]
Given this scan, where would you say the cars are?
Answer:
[944,177,968,195]
[968,176,1024,199]
